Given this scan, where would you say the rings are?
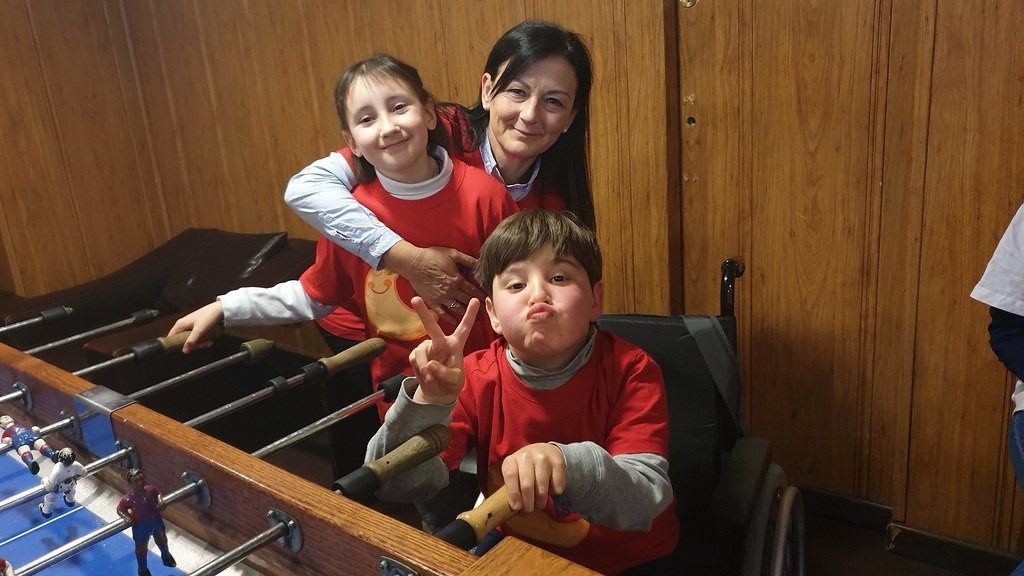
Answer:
[447,300,458,311]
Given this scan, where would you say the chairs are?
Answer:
[0,226,288,431]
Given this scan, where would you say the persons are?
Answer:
[39,447,88,519]
[116,467,177,576]
[0,415,60,475]
[362,209,680,576]
[167,52,522,536]
[283,19,599,242]
[968,203,1023,491]
